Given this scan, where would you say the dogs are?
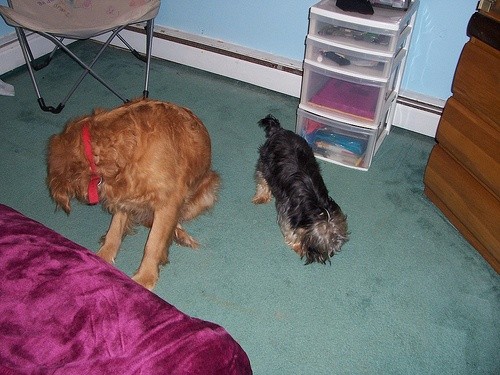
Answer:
[46,98,220,293]
[250,114,351,267]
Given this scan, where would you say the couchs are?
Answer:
[0,204,253,375]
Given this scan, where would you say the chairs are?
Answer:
[0,0,161,114]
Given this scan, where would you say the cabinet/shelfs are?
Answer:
[423,0,500,276]
[296,0,420,171]
